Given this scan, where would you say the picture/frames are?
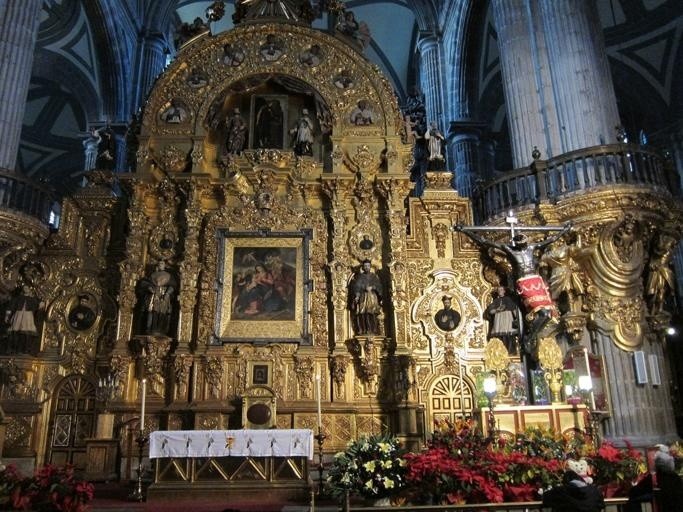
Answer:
[209,226,313,346]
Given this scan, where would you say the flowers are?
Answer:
[0,463,93,512]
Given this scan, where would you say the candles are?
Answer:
[140,378,146,430]
[315,374,321,428]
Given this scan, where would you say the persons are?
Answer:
[540,470,606,511]
[435,294,461,331]
[68,295,95,330]
[5,281,46,356]
[351,260,384,335]
[628,448,683,511]
[90,0,451,173]
[454,218,683,357]
[133,266,156,336]
[144,257,176,334]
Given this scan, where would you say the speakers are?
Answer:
[634,350,648,385]
[648,354,661,386]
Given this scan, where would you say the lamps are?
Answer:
[483,379,497,439]
[577,375,596,442]
